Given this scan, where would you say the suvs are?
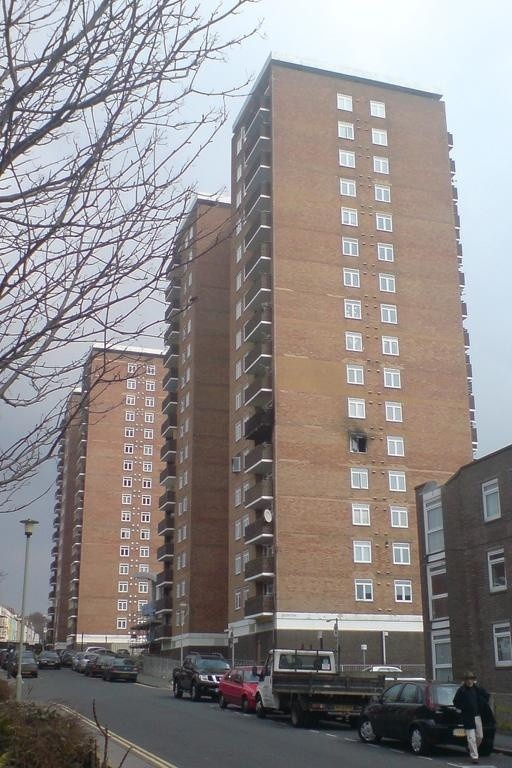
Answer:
[173,651,232,701]
[359,679,497,758]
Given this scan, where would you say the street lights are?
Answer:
[15,517,40,703]
[326,617,341,673]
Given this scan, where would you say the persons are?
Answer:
[453,672,490,764]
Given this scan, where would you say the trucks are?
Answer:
[252,647,387,728]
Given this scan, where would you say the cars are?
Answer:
[362,666,402,673]
[216,664,265,713]
[0,646,138,683]
